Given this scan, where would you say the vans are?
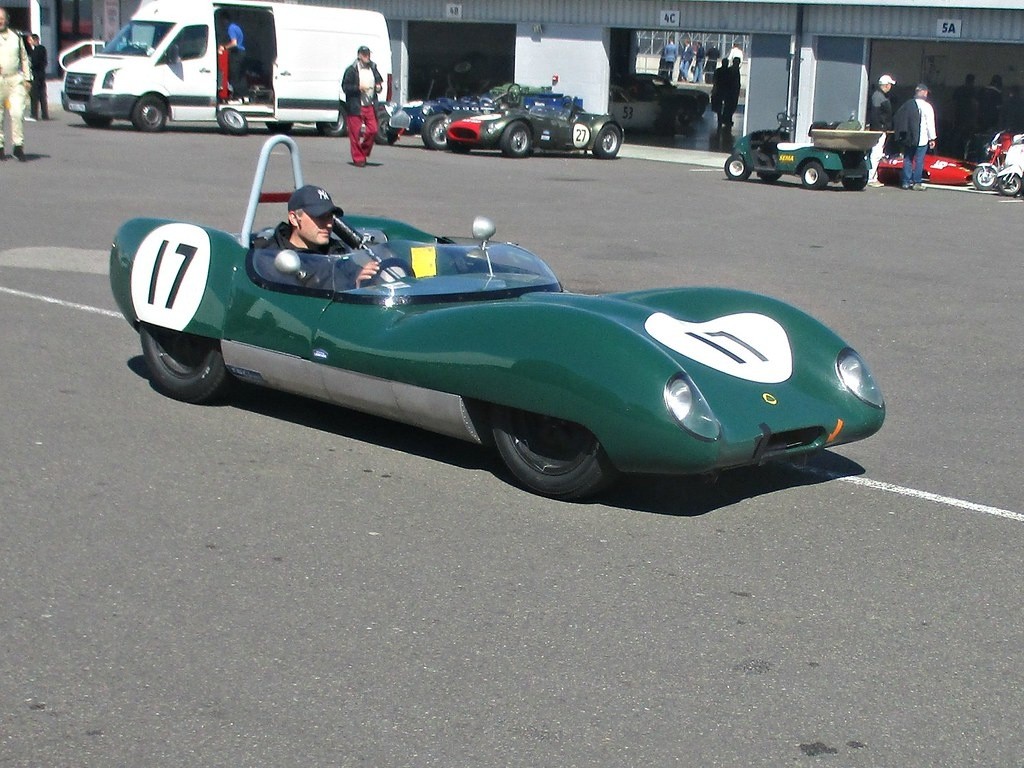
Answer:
[60,0,394,132]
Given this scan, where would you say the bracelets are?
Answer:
[224,46,226,50]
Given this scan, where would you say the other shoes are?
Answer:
[0,147,7,161]
[902,183,926,191]
[11,146,26,163]
[228,96,250,105]
[867,180,884,188]
[354,160,365,167]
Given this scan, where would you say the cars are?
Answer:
[635,73,710,120]
[609,83,692,131]
[446,84,625,159]
[877,152,979,185]
[375,83,583,151]
[110,134,885,502]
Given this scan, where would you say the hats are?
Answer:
[358,45,371,55]
[878,75,896,84]
[914,84,931,93]
[288,185,344,218]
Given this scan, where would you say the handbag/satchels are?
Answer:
[375,102,389,120]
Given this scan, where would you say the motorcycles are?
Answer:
[972,130,1013,191]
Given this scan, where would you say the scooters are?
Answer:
[995,134,1024,196]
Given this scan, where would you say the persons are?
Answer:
[257,185,389,291]
[892,83,937,191]
[0,8,32,161]
[953,74,978,159]
[728,43,743,67]
[689,40,705,83]
[679,38,694,82]
[24,34,50,122]
[711,57,741,129]
[867,74,895,187]
[341,46,384,167]
[705,42,721,84]
[974,74,1005,131]
[662,38,678,82]
[219,11,249,105]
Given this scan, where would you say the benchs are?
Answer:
[529,104,573,120]
[255,224,401,283]
